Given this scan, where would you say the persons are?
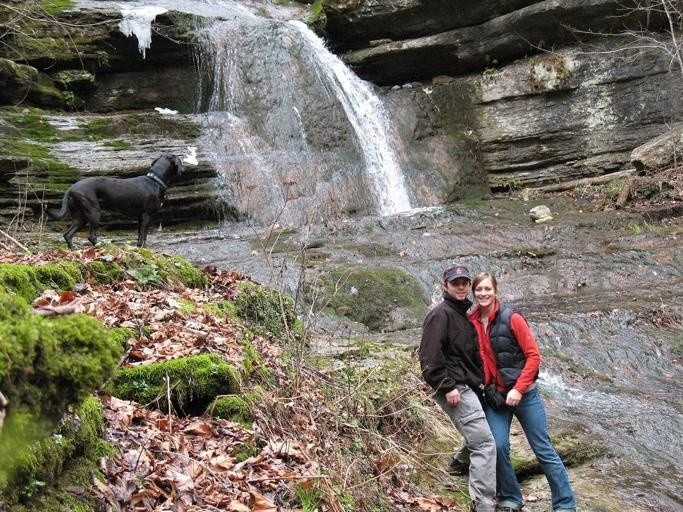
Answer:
[417,262,500,512]
[465,270,579,512]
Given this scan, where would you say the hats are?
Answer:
[443,268,471,283]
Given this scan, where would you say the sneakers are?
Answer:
[497,504,577,512]
[445,458,469,473]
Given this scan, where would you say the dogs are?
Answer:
[42,153,186,252]
